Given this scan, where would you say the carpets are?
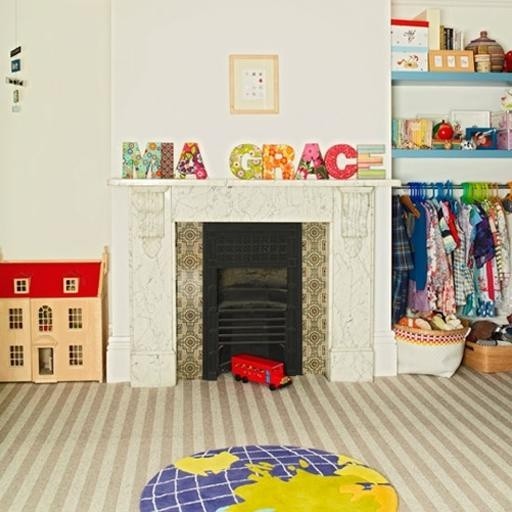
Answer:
[139,443,401,512]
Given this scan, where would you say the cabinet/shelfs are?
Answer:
[390,69,512,160]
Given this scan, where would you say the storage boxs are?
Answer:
[391,7,475,73]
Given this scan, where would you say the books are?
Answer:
[412,8,465,51]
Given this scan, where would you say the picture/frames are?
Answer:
[228,53,281,116]
